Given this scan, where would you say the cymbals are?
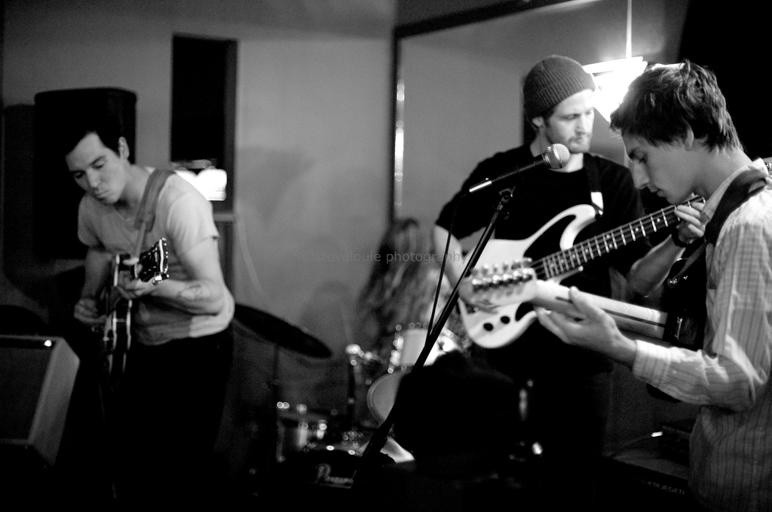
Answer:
[230,303,332,360]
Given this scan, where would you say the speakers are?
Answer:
[31,88,136,260]
[0,334,81,469]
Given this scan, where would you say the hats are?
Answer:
[523,55,595,120]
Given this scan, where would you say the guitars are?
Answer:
[455,156,772,350]
[94,239,170,376]
[472,258,708,402]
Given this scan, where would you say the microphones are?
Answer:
[469,143,570,193]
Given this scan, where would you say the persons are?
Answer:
[58,113,238,500]
[432,54,711,468]
[535,60,772,512]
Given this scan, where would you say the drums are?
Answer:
[366,323,464,425]
[274,400,328,462]
[283,430,414,491]
